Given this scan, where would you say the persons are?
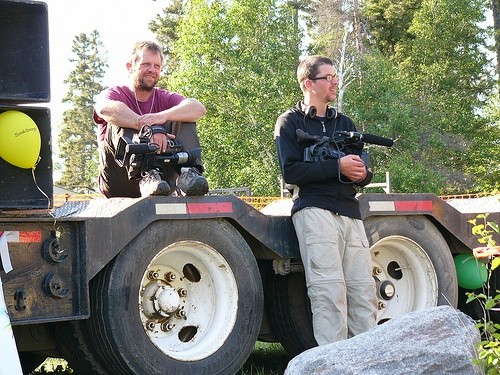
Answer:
[274,56,379,346]
[92,41,208,198]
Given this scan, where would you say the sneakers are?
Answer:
[136,166,211,197]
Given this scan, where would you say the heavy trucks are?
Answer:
[0,0,500,375]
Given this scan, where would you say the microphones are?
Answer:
[336,130,393,146]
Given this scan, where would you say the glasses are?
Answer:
[309,74,342,82]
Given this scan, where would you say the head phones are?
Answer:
[301,101,337,120]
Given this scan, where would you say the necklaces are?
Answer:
[134,89,155,118]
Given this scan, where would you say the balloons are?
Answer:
[454,253,488,289]
[0,110,41,168]
